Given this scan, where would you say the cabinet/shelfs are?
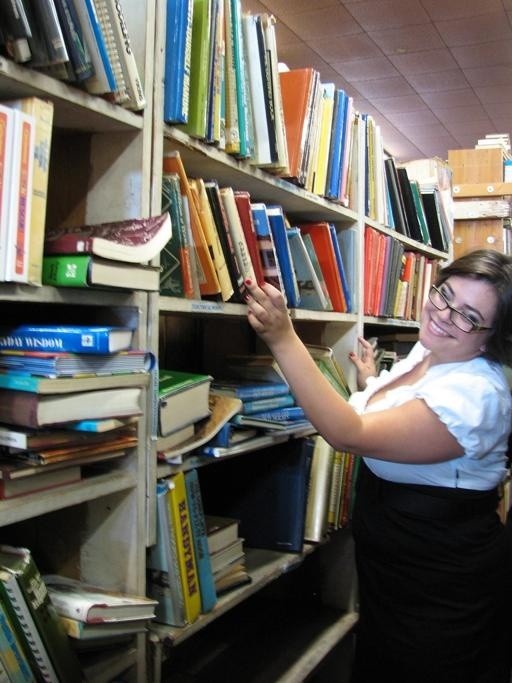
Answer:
[155,17,362,683]
[126,279,143,283]
[363,216,454,629]
[0,0,154,676]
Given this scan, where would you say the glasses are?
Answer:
[426,283,496,333]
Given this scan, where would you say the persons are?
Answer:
[242,249,512,682]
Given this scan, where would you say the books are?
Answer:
[162,150,353,313]
[0,0,146,111]
[156,342,353,465]
[368,336,399,375]
[0,324,156,500]
[363,112,454,253]
[475,133,512,161]
[0,541,160,683]
[364,226,449,321]
[0,91,173,290]
